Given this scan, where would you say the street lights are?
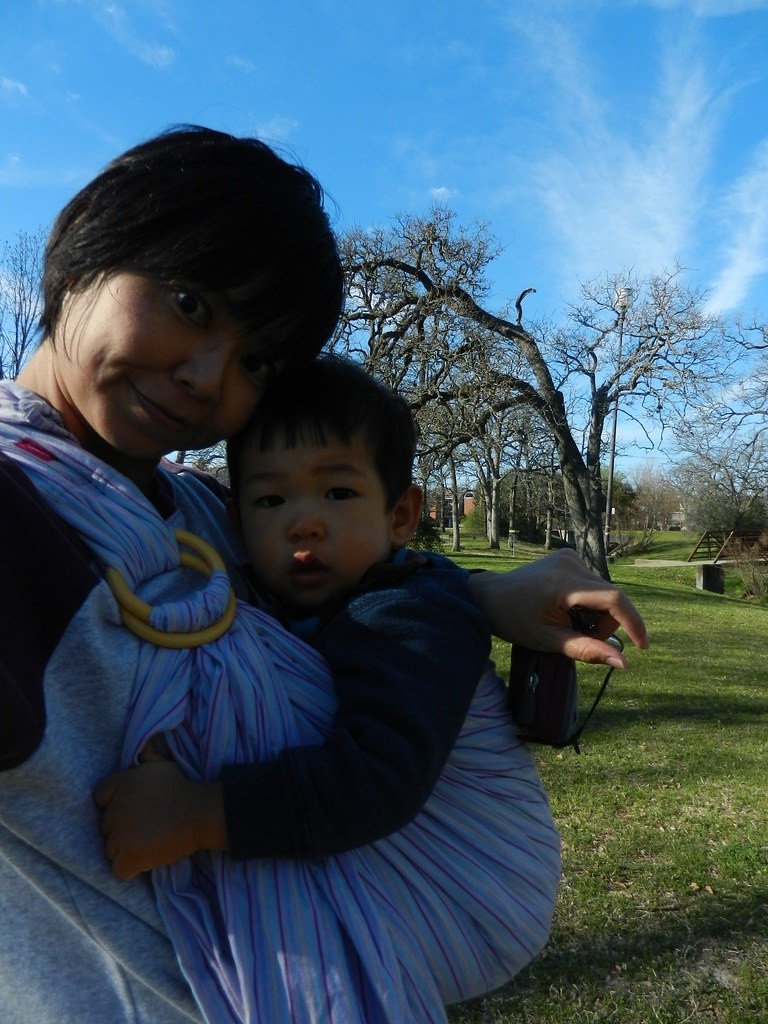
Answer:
[602,285,636,555]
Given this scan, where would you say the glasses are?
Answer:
[509,627,623,747]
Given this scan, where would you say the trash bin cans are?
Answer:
[508,527,520,547]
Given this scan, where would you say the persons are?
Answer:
[89,354,560,1004]
[0,121,652,1023]
